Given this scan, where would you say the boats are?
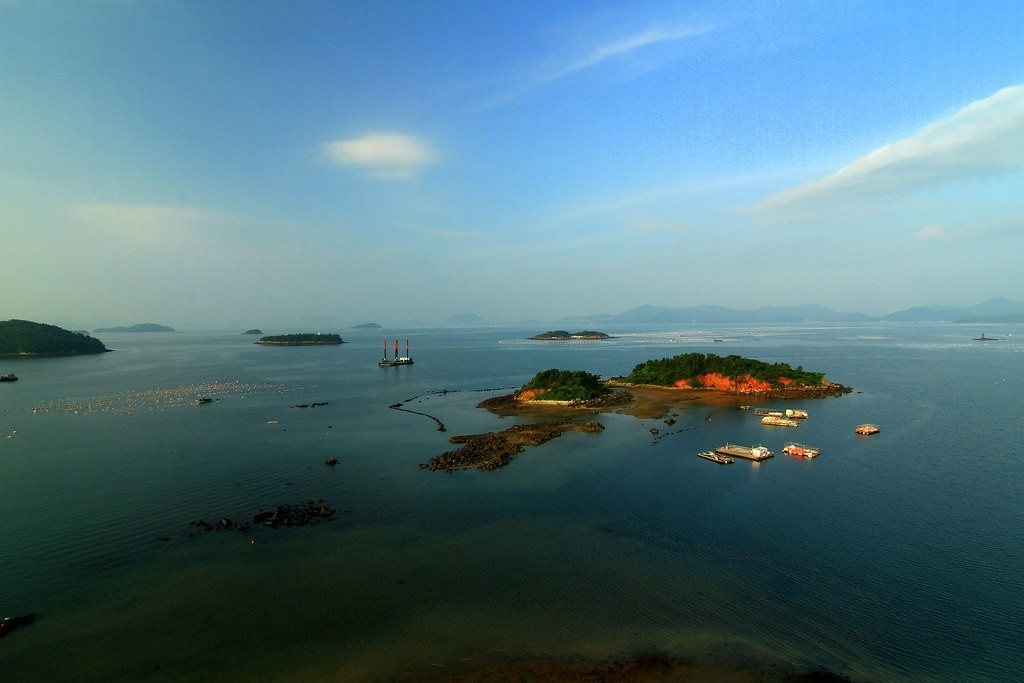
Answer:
[761,416,799,427]
[698,451,735,465]
[754,409,783,418]
[856,423,881,435]
[715,443,775,461]
[781,443,820,458]
[785,408,808,419]
[378,338,415,367]
